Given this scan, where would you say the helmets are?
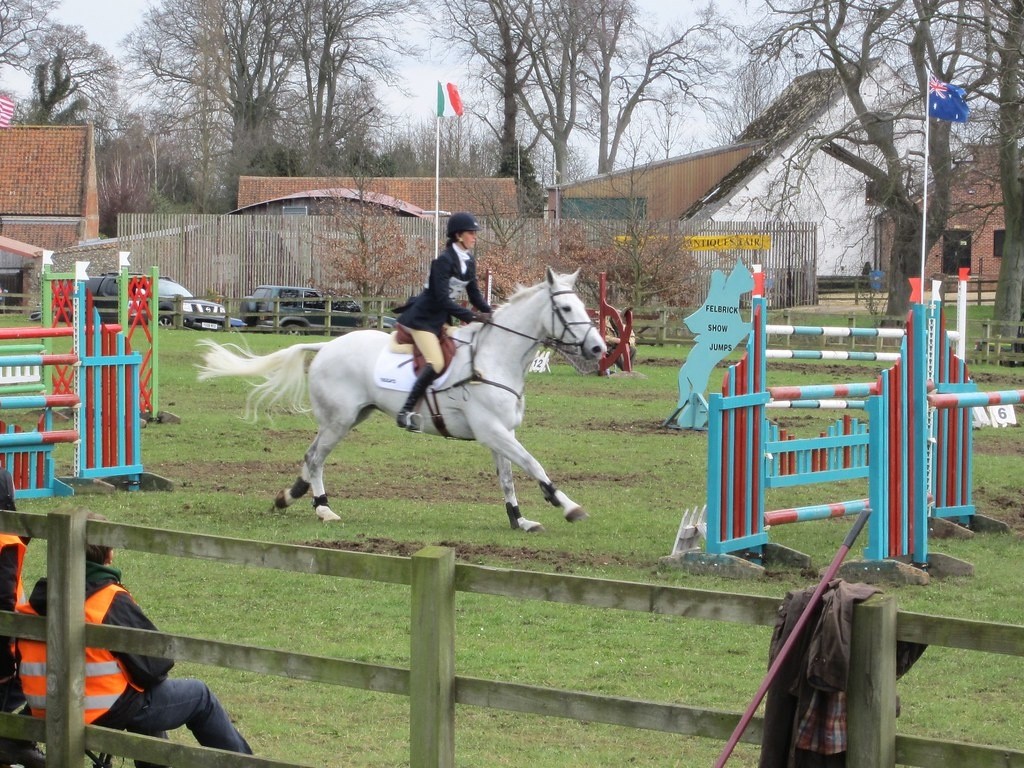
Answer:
[448,213,483,233]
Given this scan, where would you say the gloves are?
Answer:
[475,311,490,323]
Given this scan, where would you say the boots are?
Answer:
[398,363,441,430]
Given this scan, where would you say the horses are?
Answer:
[191,264,607,534]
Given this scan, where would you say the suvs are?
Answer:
[54,272,225,331]
[240,285,397,337]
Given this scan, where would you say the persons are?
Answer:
[0,462,253,768]
[392,212,493,431]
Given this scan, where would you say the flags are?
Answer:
[447,82,463,116]
[0,92,14,128]
[437,81,444,118]
[929,73,969,122]
